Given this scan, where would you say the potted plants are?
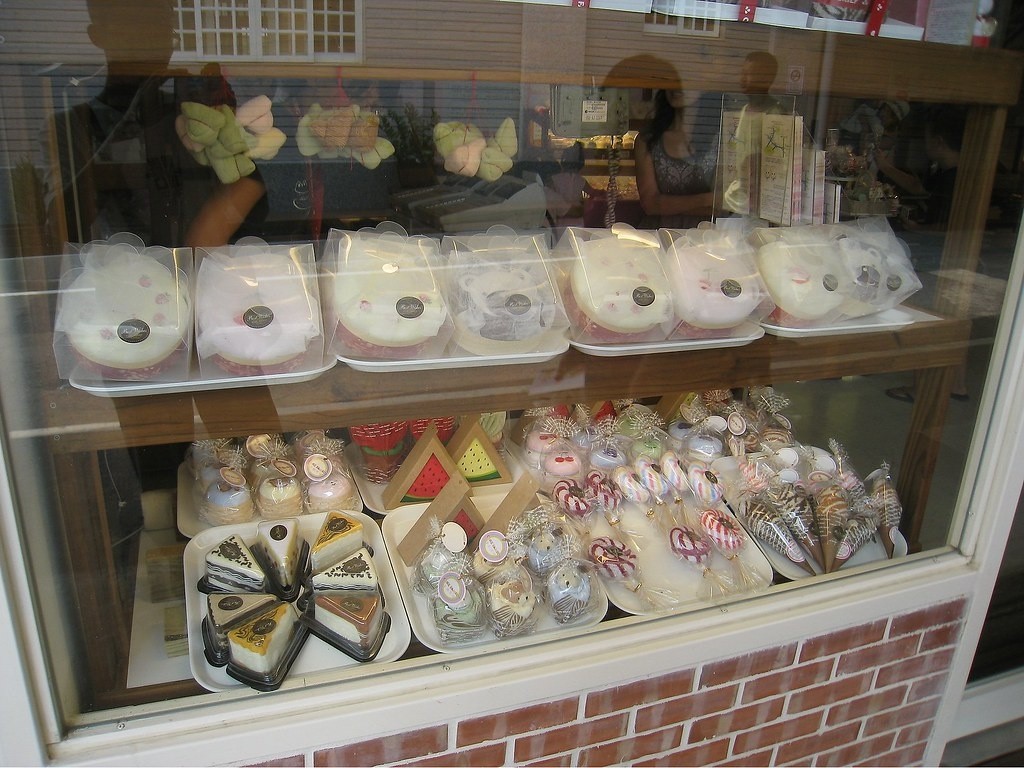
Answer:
[378,102,442,189]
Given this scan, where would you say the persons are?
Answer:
[183,78,403,247]
[839,98,973,402]
[43,1,286,490]
[546,58,751,232]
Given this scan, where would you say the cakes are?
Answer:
[59,232,915,375]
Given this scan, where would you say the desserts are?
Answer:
[187,402,903,680]
[175,95,636,198]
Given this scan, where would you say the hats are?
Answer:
[886,99,910,121]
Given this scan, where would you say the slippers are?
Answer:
[885,385,917,403]
[951,391,971,401]
